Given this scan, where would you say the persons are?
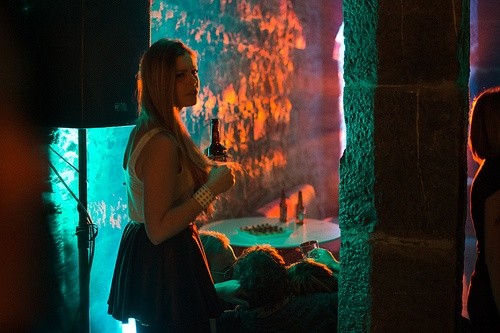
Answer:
[106,36,242,332]
[214,247,339,332]
[467,84,500,333]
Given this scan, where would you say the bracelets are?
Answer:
[192,183,217,210]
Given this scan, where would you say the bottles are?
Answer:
[207,119,227,163]
[280,188,287,223]
[295,190,304,225]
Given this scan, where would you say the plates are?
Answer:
[235,224,295,238]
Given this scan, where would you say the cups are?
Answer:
[299,240,319,259]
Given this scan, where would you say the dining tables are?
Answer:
[199,216,343,264]
